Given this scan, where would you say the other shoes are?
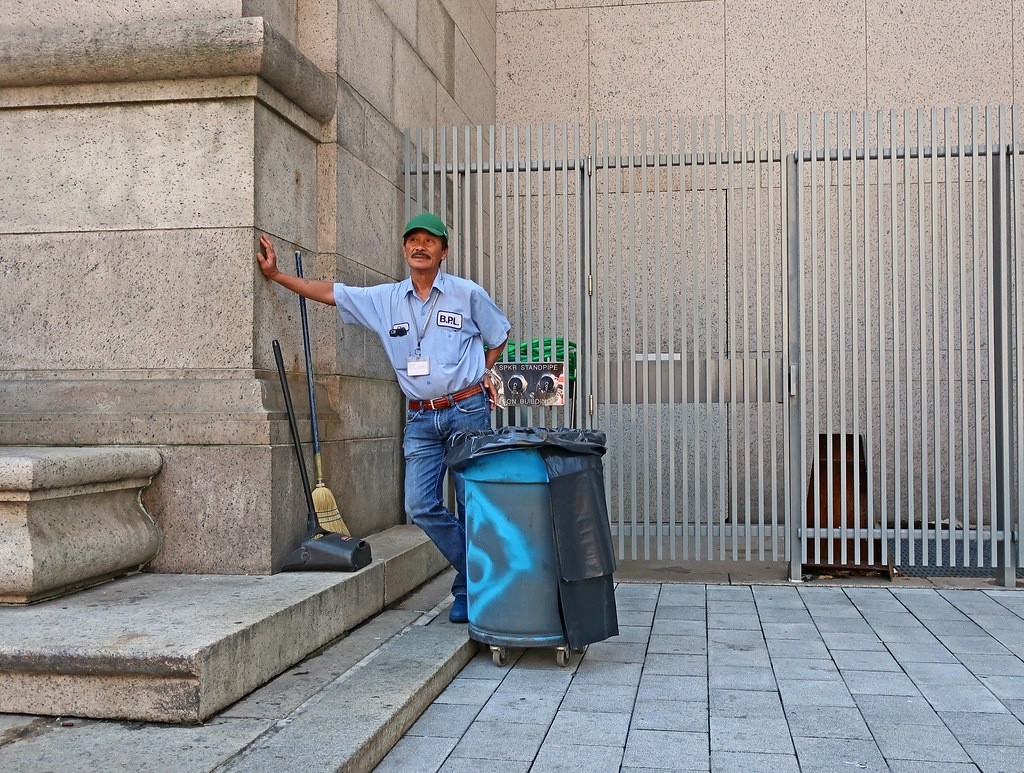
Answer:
[449,594,471,623]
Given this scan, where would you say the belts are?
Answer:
[408,383,484,410]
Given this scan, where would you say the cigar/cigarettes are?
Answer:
[489,399,504,410]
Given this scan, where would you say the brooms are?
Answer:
[294,250,352,537]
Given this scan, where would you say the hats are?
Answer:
[401,212,449,241]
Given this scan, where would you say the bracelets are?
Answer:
[485,368,493,377]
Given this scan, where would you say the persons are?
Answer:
[257,211,511,622]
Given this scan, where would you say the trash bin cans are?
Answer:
[453,426,620,670]
